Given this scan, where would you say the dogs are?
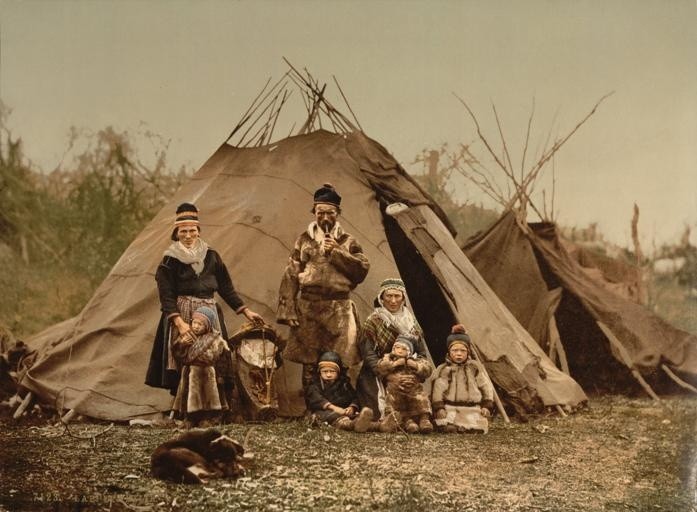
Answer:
[150,427,258,485]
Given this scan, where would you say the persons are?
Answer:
[302,350,402,434]
[144,202,266,425]
[273,184,370,416]
[168,306,233,419]
[355,277,427,421]
[376,333,432,432]
[429,323,494,434]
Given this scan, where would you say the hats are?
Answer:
[313,183,341,208]
[190,306,215,327]
[317,352,341,376]
[375,278,406,309]
[173,203,199,228]
[445,324,470,353]
[392,332,418,357]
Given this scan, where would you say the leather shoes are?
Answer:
[404,418,418,434]
[419,419,433,432]
[378,410,400,433]
[353,407,372,432]
